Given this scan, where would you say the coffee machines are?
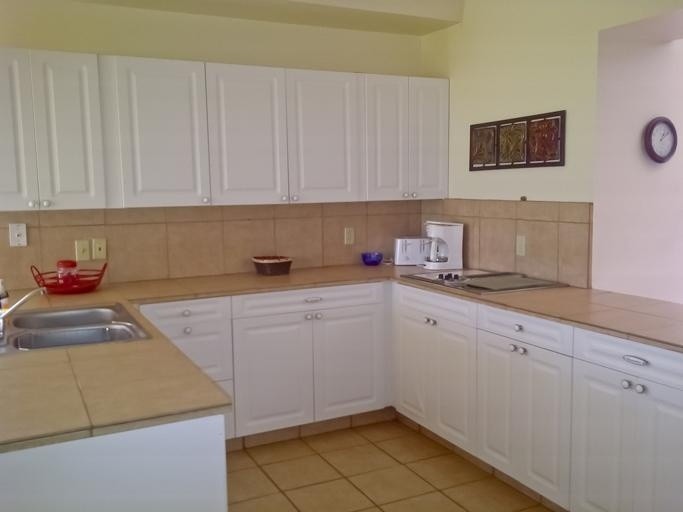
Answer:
[422,220,464,271]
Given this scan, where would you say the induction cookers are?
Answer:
[415,269,569,293]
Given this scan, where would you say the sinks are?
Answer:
[13,302,135,328]
[13,324,151,351]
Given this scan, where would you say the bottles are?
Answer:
[56,259,78,289]
[0,278,10,327]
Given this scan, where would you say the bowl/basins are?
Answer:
[361,251,383,266]
[250,255,293,276]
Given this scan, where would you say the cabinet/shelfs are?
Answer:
[108,54,212,208]
[393,276,480,468]
[229,279,394,449]
[0,46,109,213]
[135,290,235,454]
[205,61,365,207]
[365,72,450,202]
[474,302,574,512]
[571,319,683,512]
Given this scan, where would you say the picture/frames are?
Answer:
[468,110,566,172]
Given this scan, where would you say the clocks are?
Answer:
[644,117,678,163]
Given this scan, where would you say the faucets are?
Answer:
[0,286,47,340]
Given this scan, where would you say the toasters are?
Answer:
[394,235,430,266]
